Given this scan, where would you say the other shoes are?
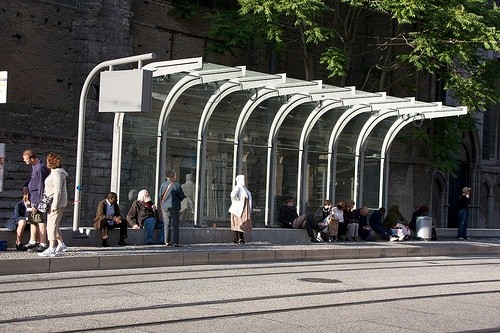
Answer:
[233,239,246,244]
[310,236,398,243]
[15,242,47,252]
[101,242,109,247]
[166,242,182,247]
[118,241,126,245]
[458,237,470,241]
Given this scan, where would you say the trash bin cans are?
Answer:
[416,216,432,241]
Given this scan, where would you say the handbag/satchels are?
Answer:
[158,204,163,220]
[28,211,44,224]
[37,194,53,214]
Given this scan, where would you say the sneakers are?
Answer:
[38,247,60,257]
[55,243,68,253]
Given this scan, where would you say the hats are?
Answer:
[462,187,471,192]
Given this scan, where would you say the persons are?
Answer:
[92,192,128,247]
[409,205,437,240]
[159,169,186,247]
[23,150,50,251]
[4,185,33,250]
[228,175,253,243]
[456,187,472,240]
[126,189,164,245]
[126,190,138,213]
[180,174,196,227]
[343,200,359,241]
[279,196,332,243]
[37,153,68,257]
[369,207,405,242]
[351,207,371,240]
[384,205,410,239]
[332,201,347,241]
[314,200,332,241]
